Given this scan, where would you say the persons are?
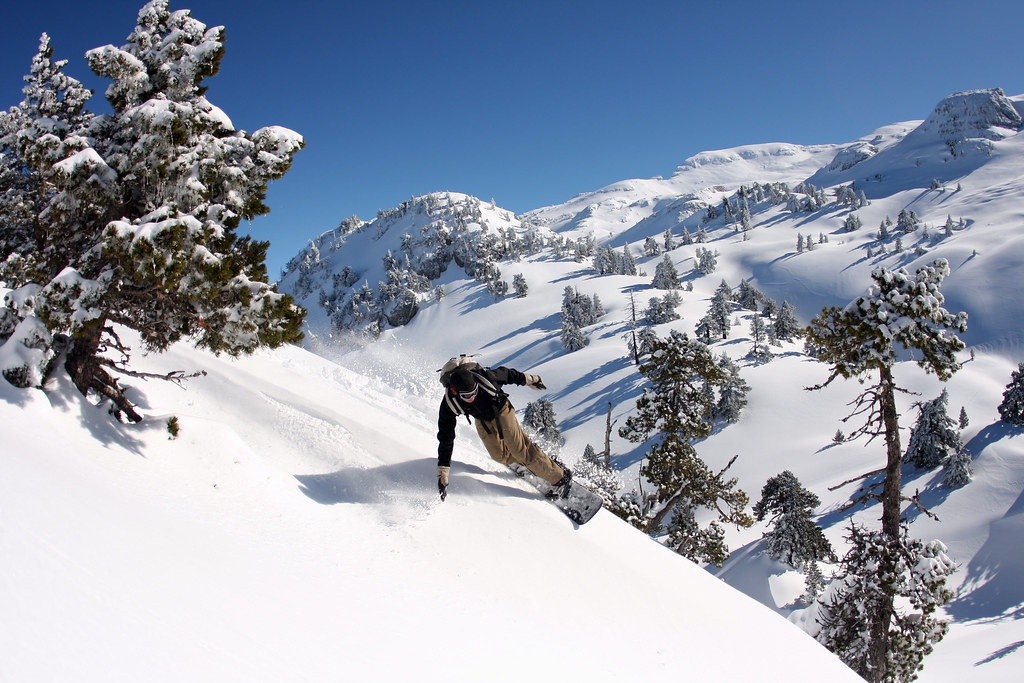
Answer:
[437,357,574,498]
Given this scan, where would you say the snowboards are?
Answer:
[509,462,604,525]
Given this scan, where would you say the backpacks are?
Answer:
[439,356,500,416]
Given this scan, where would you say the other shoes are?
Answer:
[545,471,573,500]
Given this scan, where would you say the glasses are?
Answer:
[460,383,479,402]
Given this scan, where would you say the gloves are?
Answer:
[437,465,450,502]
[525,375,547,391]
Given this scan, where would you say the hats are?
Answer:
[450,370,475,392]
